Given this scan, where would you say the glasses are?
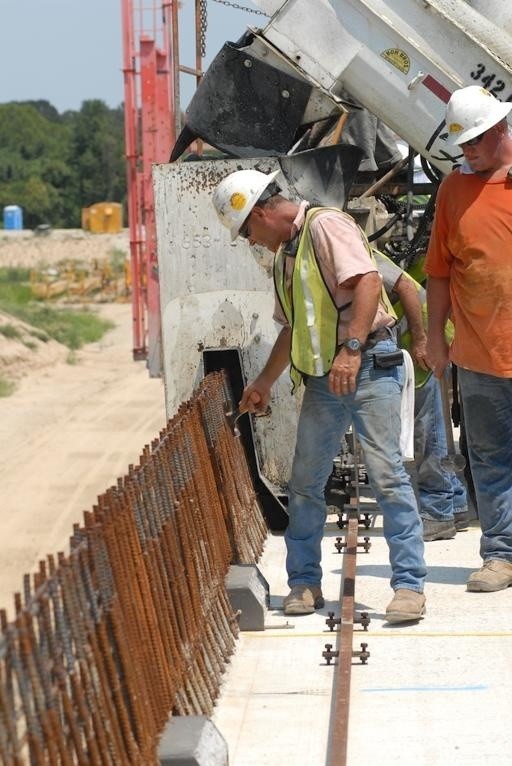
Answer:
[239,222,248,238]
[466,133,484,145]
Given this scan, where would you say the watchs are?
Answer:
[343,337,367,356]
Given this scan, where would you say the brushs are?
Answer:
[224,392,262,438]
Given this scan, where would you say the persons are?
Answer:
[372,248,473,540]
[213,170,425,623]
[426,85,512,592]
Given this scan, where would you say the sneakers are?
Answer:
[421,511,469,542]
[467,556,511,592]
[282,584,324,617]
[384,589,426,623]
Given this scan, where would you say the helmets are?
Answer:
[444,85,511,146]
[212,169,280,240]
